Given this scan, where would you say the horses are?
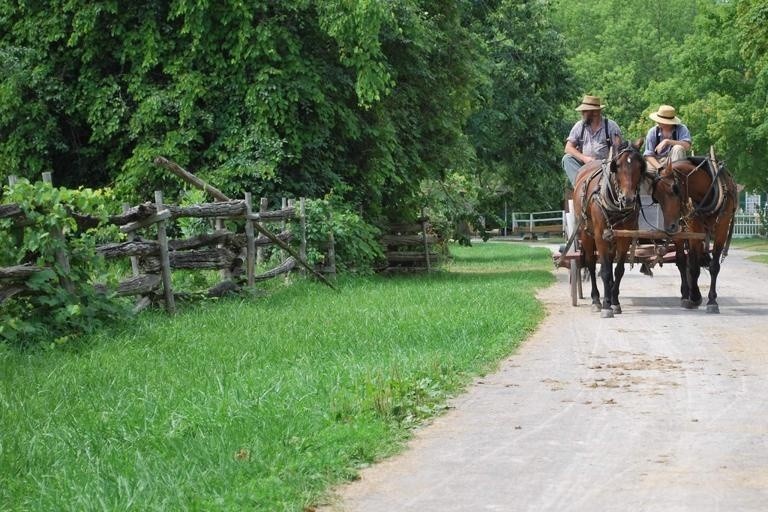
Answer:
[643,154,738,315]
[571,132,646,320]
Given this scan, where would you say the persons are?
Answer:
[561,94,623,191]
[453,189,474,247]
[644,104,692,218]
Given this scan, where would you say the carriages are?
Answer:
[553,130,741,320]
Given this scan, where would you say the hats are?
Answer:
[575,96,606,111]
[649,104,681,125]
